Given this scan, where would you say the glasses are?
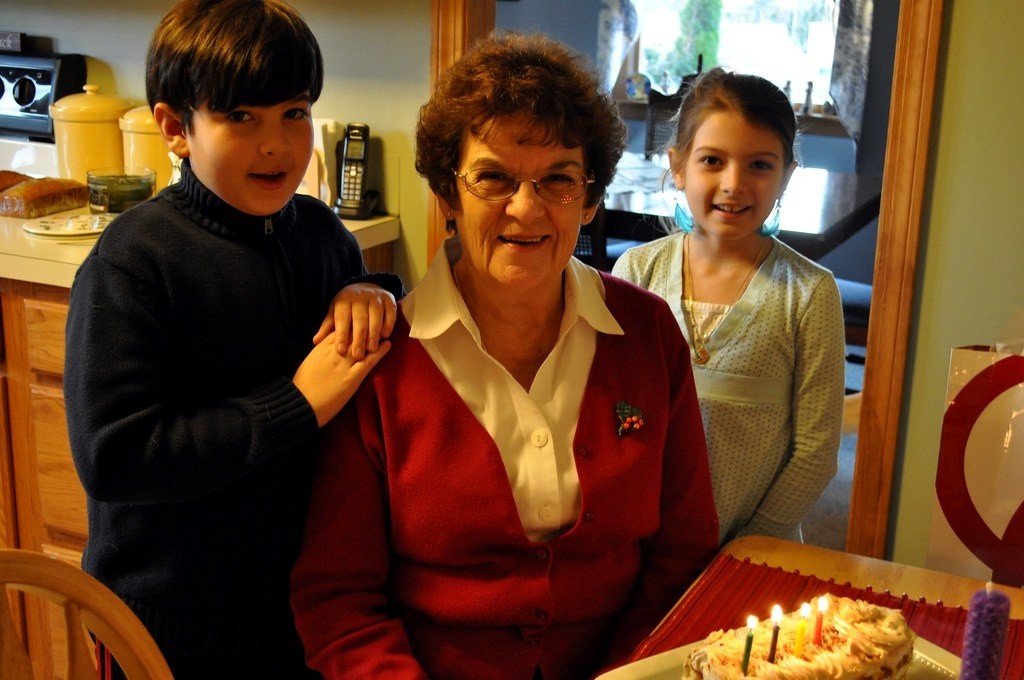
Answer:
[452,166,596,203]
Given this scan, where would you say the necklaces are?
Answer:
[453,263,488,355]
[684,232,768,365]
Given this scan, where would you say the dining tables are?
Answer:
[599,533,1024,679]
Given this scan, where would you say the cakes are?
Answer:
[681,593,918,680]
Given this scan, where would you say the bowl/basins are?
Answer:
[86,169,157,214]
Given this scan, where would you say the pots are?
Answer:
[50,84,134,184]
[119,105,173,197]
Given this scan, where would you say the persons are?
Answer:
[63,3,397,680]
[613,66,843,540]
[290,28,720,679]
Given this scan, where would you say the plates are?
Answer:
[23,213,117,235]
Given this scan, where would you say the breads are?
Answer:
[1,169,88,218]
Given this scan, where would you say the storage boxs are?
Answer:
[48,83,136,186]
[118,104,173,197]
[87,168,156,214]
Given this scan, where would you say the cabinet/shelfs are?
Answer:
[0,243,417,679]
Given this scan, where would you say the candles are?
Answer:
[740,631,750,677]
[959,582,1010,679]
[812,612,824,647]
[767,625,780,665]
[796,619,804,658]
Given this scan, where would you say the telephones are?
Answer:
[341,122,369,200]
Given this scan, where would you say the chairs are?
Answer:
[0,548,174,680]
